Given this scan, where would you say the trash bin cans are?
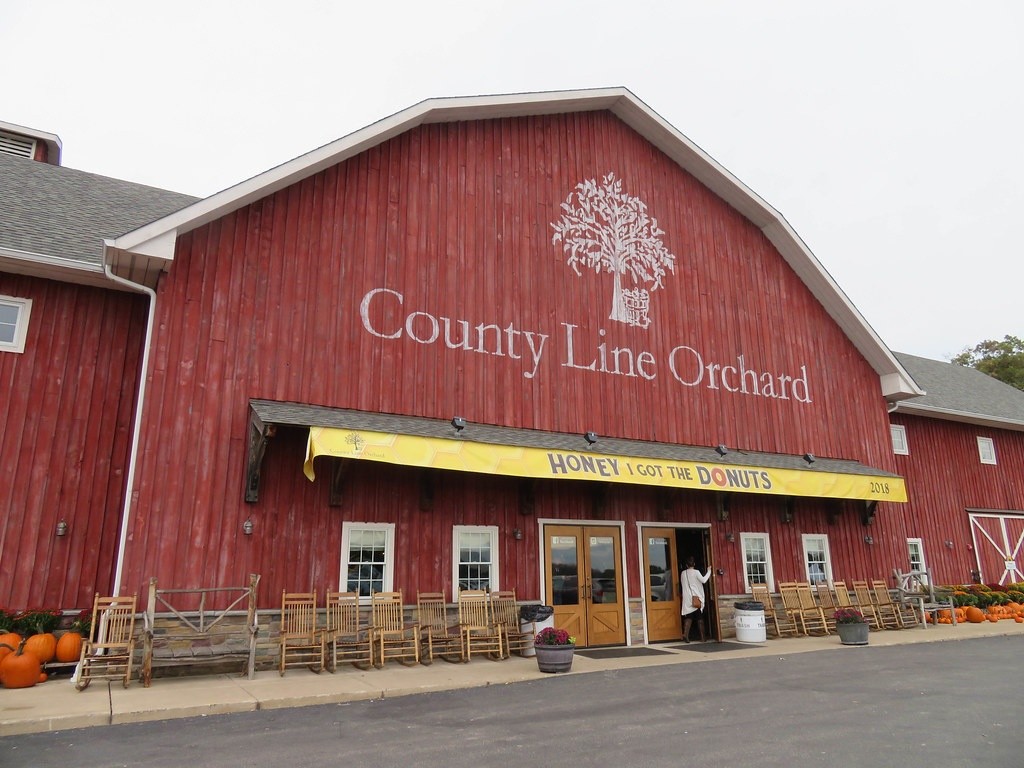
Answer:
[733,601,767,642]
[520,605,554,656]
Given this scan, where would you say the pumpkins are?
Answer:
[918,597,1024,623]
[0,623,83,688]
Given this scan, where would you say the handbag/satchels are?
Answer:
[692,596,702,608]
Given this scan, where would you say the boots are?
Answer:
[698,620,706,642]
[682,619,692,643]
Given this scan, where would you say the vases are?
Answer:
[535,644,575,673]
[837,622,869,644]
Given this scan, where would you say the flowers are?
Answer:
[833,608,865,624]
[535,627,576,645]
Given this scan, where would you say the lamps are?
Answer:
[945,540,954,549]
[584,432,597,444]
[243,519,253,535]
[55,518,67,536]
[513,527,523,539]
[727,533,735,542]
[451,417,466,430]
[803,453,816,463]
[715,445,727,455]
[865,536,873,544]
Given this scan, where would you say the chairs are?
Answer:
[458,587,503,661]
[490,588,538,659]
[323,588,375,673]
[416,590,465,666]
[371,588,420,669]
[279,589,326,676]
[749,578,920,641]
[71,593,137,688]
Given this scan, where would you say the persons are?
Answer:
[561,565,578,605]
[591,555,603,604]
[681,555,711,643]
[660,568,672,601]
